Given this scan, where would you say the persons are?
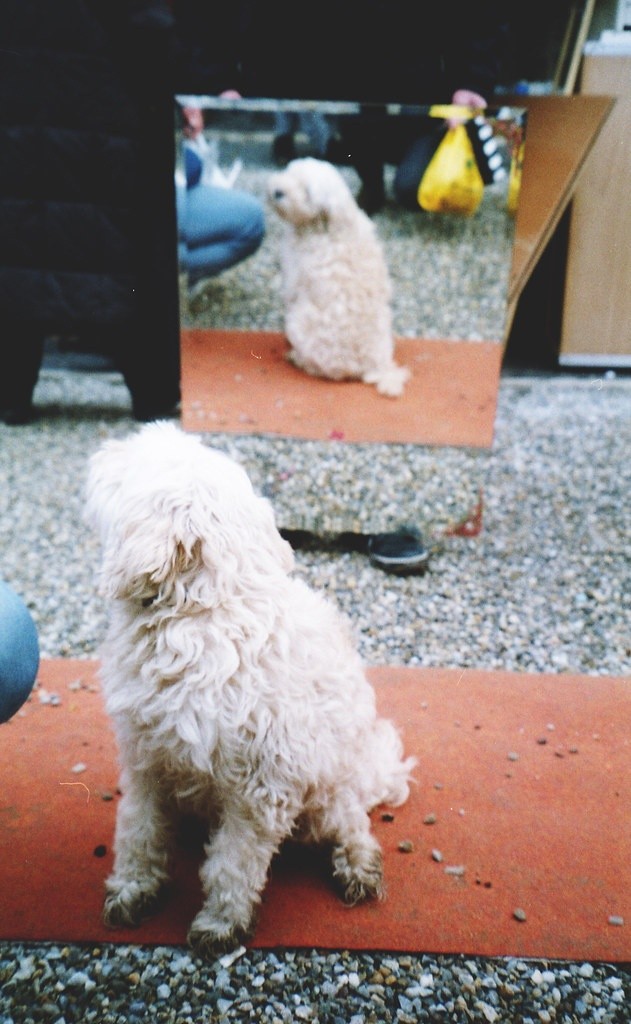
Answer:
[212,0,511,571]
[175,113,492,291]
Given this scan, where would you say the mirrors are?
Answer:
[175,91,528,450]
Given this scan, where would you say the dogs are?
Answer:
[80,418,419,949]
[267,157,411,398]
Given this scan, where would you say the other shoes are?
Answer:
[370,533,430,573]
